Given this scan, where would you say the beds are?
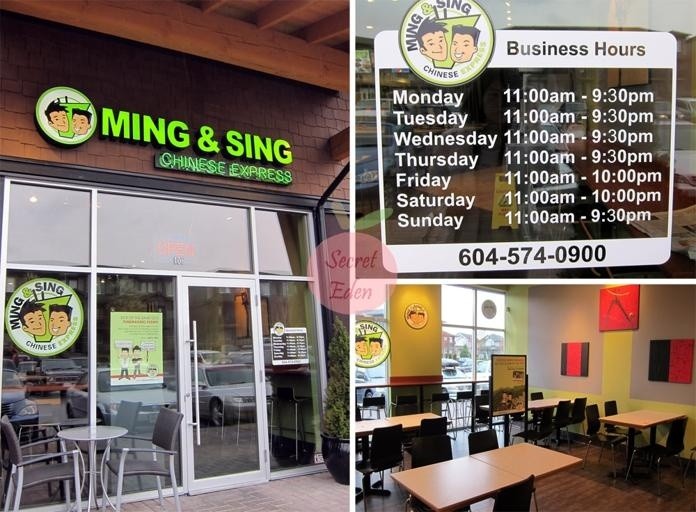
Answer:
[57,425,128,512]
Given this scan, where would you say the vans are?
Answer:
[192,364,275,428]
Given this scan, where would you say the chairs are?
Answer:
[356,424,403,512]
[402,417,447,455]
[403,436,472,512]
[360,394,388,419]
[390,395,417,416]
[584,404,627,477]
[531,392,554,431]
[554,400,571,450]
[267,371,313,462]
[491,474,535,512]
[509,412,526,432]
[108,401,142,495]
[467,429,539,512]
[100,407,183,512]
[456,391,473,433]
[626,416,688,496]
[511,406,554,448]
[425,393,456,440]
[0,415,82,512]
[603,400,642,454]
[569,397,587,442]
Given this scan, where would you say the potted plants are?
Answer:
[319,316,350,485]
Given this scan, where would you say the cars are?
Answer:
[18,361,38,373]
[4,359,16,371]
[66,368,177,428]
[1,368,39,443]
[71,357,89,373]
[13,355,31,366]
[191,350,223,364]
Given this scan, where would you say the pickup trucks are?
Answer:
[440,365,487,401]
[41,358,88,384]
